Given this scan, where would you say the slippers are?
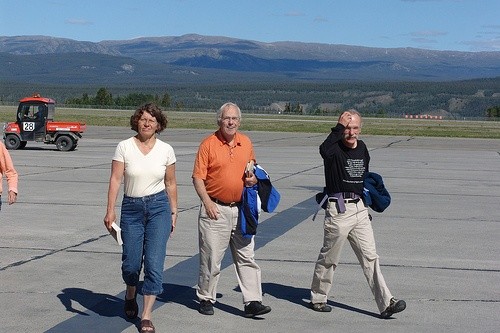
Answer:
[125,289,138,319]
[140,319,155,333]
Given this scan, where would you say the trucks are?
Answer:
[2,94,86,151]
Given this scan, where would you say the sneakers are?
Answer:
[381,298,406,319]
[244,301,271,318]
[311,302,332,312]
[199,300,214,315]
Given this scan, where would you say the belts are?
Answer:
[209,196,240,208]
[329,198,360,204]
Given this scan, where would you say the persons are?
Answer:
[104,104,178,333]
[308,109,406,318]
[192,101,271,317]
[0,141,18,210]
[34,105,44,118]
